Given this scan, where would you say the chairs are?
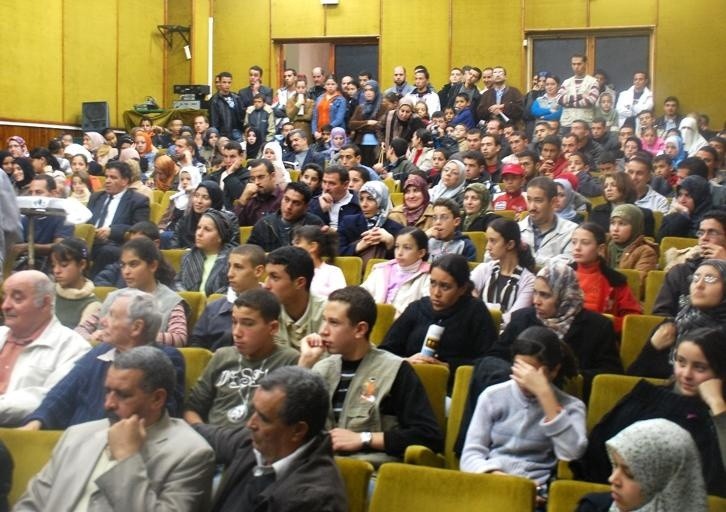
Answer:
[1,429,68,509]
[445,365,584,482]
[335,454,375,509]
[586,374,672,436]
[366,463,536,510]
[177,346,215,402]
[544,478,725,510]
[1,109,724,350]
[401,364,450,467]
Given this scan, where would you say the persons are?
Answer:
[2,107,725,510]
[202,53,683,169]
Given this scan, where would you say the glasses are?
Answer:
[695,230,719,237]
[431,216,449,220]
[685,274,720,284]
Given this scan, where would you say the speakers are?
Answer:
[81,101,108,131]
